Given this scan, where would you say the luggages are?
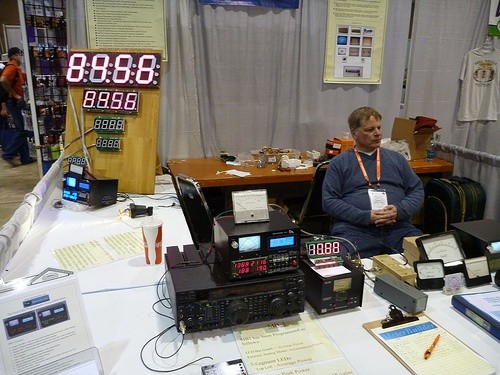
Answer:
[423,176,486,233]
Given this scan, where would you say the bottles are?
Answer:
[424,141,437,163]
[324,139,342,160]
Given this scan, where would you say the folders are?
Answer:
[451,287,500,341]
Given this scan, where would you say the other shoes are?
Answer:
[22,157,37,165]
[3,157,19,166]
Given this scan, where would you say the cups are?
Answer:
[257,156,268,168]
[140,219,163,265]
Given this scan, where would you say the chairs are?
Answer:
[284,161,332,235]
[176,174,213,244]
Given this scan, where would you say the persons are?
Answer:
[322,106,424,259]
[0,47,37,165]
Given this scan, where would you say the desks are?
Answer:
[230,253,500,375]
[0,174,246,374]
[166,156,455,186]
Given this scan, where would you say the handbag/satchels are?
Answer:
[7,97,25,110]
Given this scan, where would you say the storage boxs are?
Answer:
[263,149,300,163]
[325,138,354,156]
[391,117,441,159]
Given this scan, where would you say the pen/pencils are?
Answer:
[424,334,441,360]
